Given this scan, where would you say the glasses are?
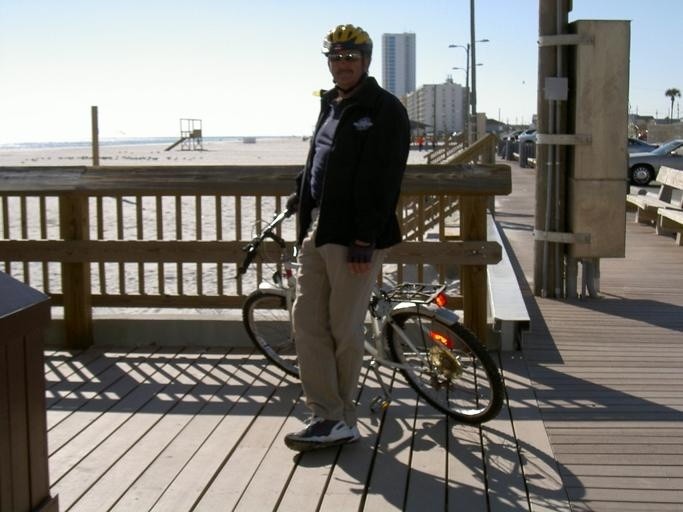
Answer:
[329,54,361,62]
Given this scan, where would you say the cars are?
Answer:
[499,129,536,146]
[627,136,659,159]
[628,140,683,186]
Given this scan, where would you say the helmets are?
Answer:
[322,24,372,52]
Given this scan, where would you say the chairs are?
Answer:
[626,166,683,246]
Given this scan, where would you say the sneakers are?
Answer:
[285,419,359,450]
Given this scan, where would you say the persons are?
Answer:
[279,18,414,452]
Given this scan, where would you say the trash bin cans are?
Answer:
[519,142,528,167]
[505,141,513,161]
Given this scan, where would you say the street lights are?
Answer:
[448,39,488,148]
[451,63,482,146]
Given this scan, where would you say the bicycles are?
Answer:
[236,191,503,424]
